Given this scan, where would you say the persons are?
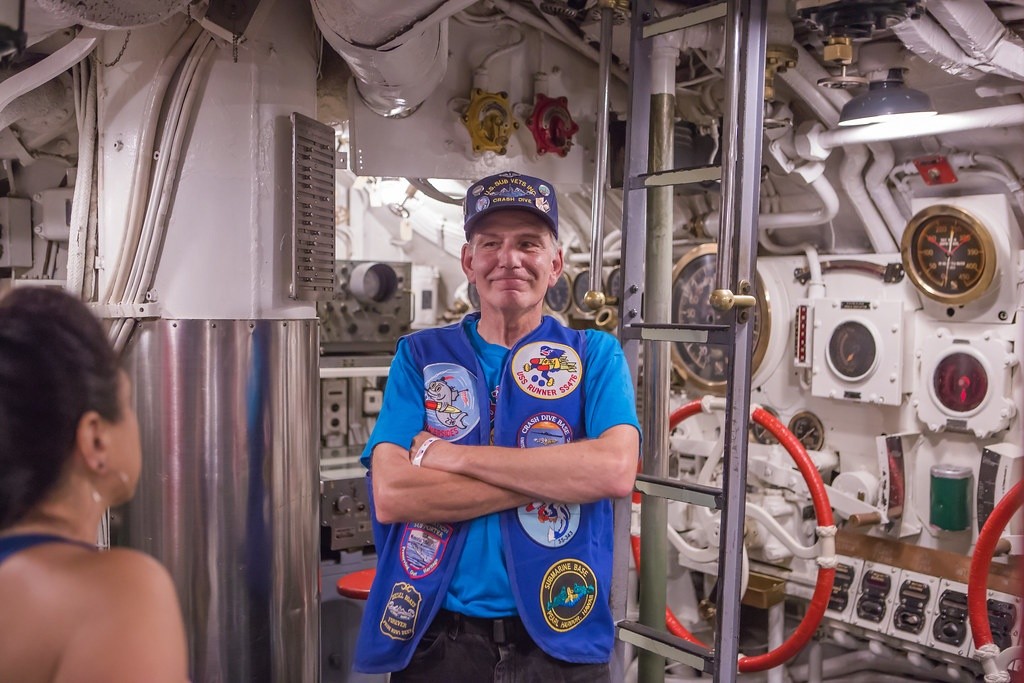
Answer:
[353,172,642,683]
[0,287,190,682]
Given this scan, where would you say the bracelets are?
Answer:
[413,436,442,468]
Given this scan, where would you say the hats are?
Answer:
[464,169,558,242]
